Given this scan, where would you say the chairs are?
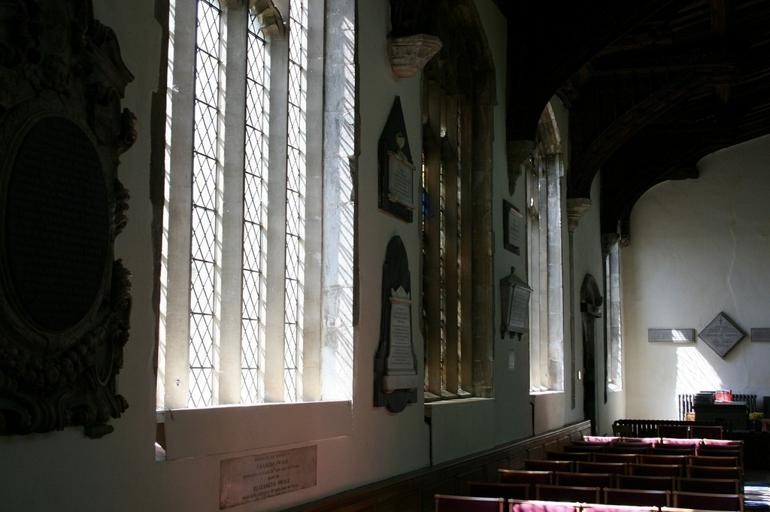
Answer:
[433,419,746,512]
